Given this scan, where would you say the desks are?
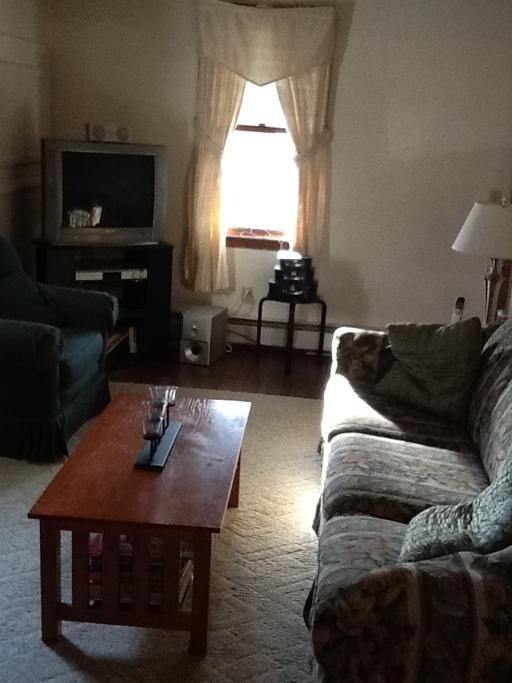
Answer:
[32,239,174,372]
[256,296,326,374]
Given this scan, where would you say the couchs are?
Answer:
[303,318,512,683]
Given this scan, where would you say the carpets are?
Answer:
[0,381,321,683]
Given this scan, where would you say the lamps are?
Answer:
[451,197,512,329]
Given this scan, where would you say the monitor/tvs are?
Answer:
[40,138,167,247]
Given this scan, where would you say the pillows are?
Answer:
[399,466,512,563]
[374,316,482,414]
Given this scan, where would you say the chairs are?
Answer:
[0,236,120,464]
